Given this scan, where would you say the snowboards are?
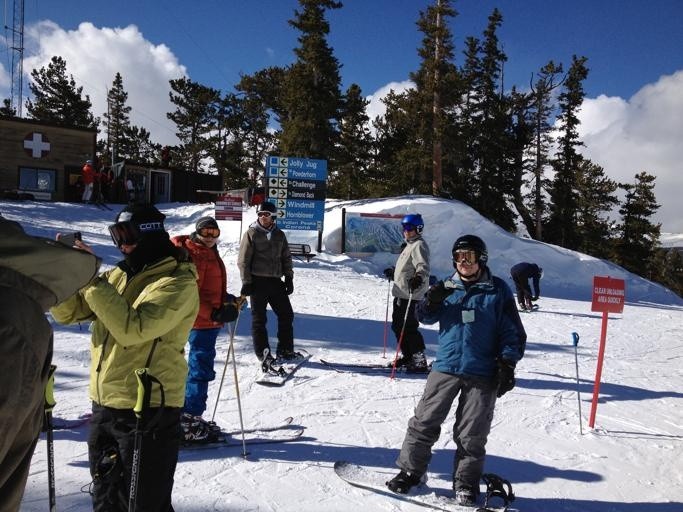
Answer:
[255,348,312,384]
[334,460,504,512]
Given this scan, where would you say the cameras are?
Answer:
[58,231,81,246]
[444,280,464,290]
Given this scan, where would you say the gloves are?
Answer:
[226,295,246,312]
[285,277,293,295]
[241,285,253,295]
[384,266,394,280]
[424,281,454,304]
[408,276,422,293]
[210,305,238,323]
[493,364,515,398]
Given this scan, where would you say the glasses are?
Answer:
[108,222,141,248]
[401,224,416,231]
[197,228,220,238]
[452,250,480,264]
[257,211,271,217]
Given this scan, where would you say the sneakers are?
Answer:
[520,299,533,309]
[277,351,304,360]
[388,469,428,493]
[262,357,285,376]
[388,351,427,371]
[180,413,221,443]
[456,487,480,506]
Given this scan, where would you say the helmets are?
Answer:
[539,267,544,280]
[401,215,424,234]
[256,202,276,220]
[452,235,487,272]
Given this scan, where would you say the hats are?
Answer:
[196,217,218,234]
[115,202,165,245]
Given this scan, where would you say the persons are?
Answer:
[510,262,544,312]
[387,234,527,506]
[383,214,431,374]
[82,160,102,204]
[171,217,247,446]
[0,210,103,512]
[237,201,302,374]
[49,202,200,512]
[127,175,136,209]
